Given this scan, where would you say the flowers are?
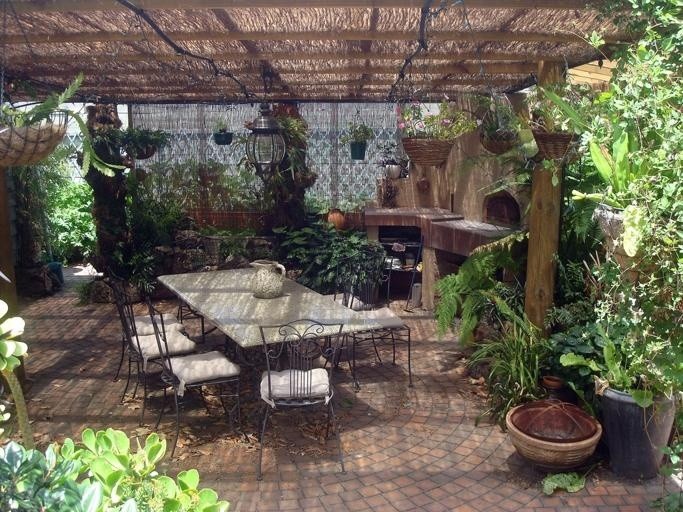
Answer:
[467,94,521,132]
[395,92,482,141]
[522,83,592,132]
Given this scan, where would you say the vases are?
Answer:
[506,403,604,473]
[533,129,577,160]
[236,236,250,248]
[543,376,564,393]
[328,207,345,230]
[481,130,518,154]
[253,236,267,248]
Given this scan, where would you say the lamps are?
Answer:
[246,67,287,191]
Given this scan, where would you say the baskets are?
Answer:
[478,130,519,157]
[529,128,578,162]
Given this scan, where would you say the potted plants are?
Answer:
[378,137,408,179]
[90,126,127,164]
[122,127,171,159]
[215,118,233,145]
[341,120,374,160]
[557,335,677,480]
[303,196,322,225]
[0,69,126,178]
[571,131,645,256]
[115,248,156,304]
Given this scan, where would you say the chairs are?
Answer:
[112,286,211,426]
[338,251,413,390]
[101,277,188,404]
[322,248,382,370]
[257,318,347,481]
[141,294,251,460]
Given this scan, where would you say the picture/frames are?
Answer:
[204,235,222,257]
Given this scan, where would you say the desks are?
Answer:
[156,265,385,437]
